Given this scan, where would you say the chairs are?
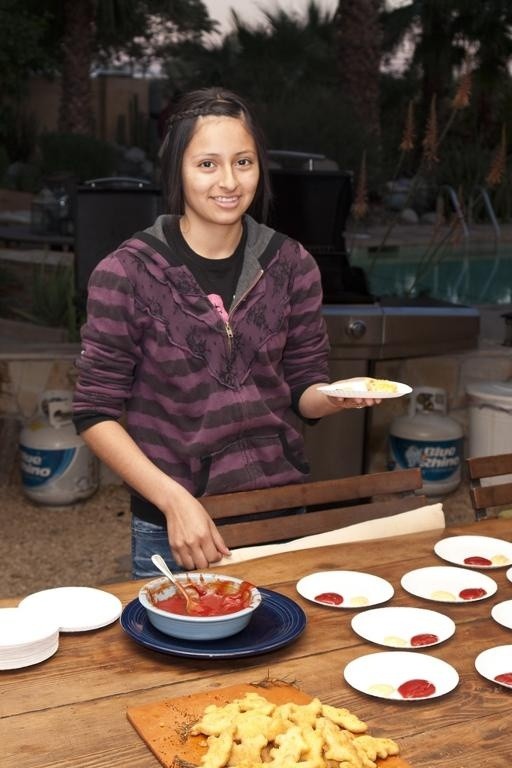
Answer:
[466,452,512,520]
[195,468,426,550]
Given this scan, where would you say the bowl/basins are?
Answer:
[139,573,260,640]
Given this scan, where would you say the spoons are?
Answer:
[148,555,205,617]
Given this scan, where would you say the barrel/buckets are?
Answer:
[464,380,511,485]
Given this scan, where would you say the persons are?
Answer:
[71,84,382,582]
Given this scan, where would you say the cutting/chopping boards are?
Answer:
[123,680,415,768]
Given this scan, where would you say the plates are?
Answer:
[397,563,496,603]
[321,380,415,401]
[20,585,121,634]
[431,532,512,571]
[0,607,61,671]
[299,566,393,609]
[117,580,308,660]
[488,593,512,632]
[339,650,461,702]
[348,606,456,649]
[474,646,510,697]
